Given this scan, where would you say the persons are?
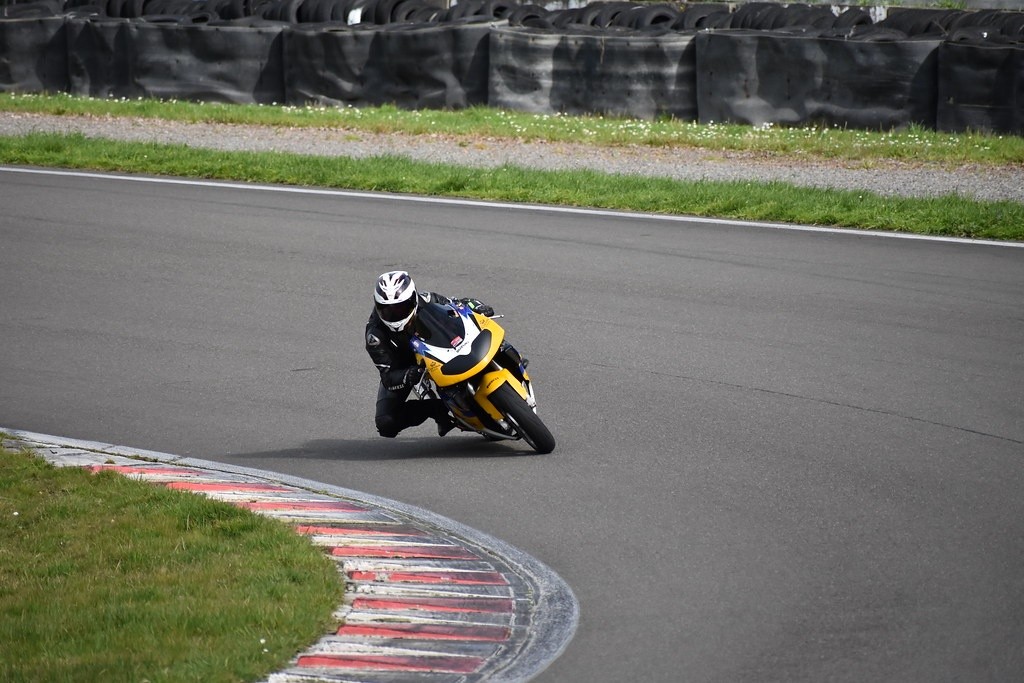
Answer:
[364,270,495,438]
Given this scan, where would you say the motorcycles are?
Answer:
[406,305,557,454]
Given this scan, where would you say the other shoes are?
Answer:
[520,358,528,368]
[433,414,451,436]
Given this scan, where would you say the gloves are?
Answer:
[407,365,424,386]
[475,304,495,317]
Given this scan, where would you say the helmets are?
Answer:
[373,270,419,333]
